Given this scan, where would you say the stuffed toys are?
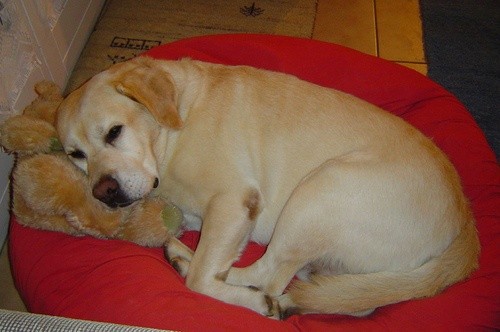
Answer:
[0,78,186,249]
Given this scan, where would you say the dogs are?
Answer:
[55,56,481,321]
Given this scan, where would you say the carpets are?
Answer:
[60,1,317,100]
